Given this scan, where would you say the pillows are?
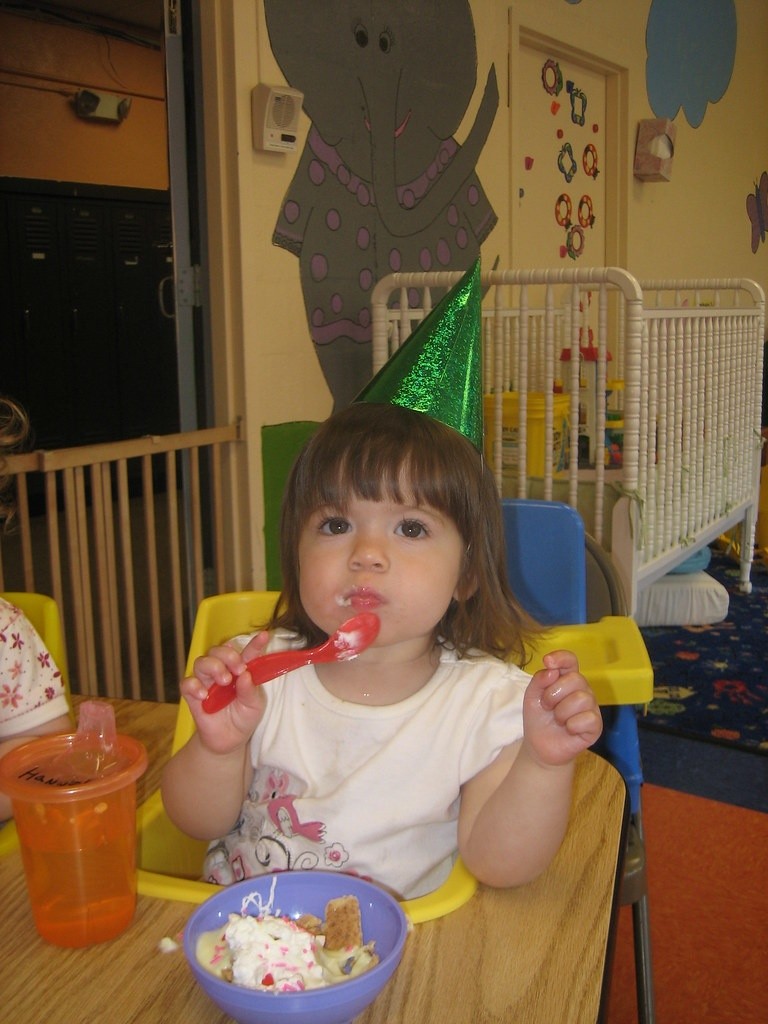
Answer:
[633,571,730,626]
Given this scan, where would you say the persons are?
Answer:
[0,594,74,825]
[159,401,604,901]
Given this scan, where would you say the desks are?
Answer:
[505,615,655,706]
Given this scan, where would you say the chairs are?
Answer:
[171,591,288,755]
[0,592,70,699]
[495,498,654,1024]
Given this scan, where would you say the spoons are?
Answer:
[202,613,381,714]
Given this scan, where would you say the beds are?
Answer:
[369,267,765,623]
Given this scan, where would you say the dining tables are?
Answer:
[0,695,625,1024]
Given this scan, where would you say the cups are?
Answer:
[0,701,149,948]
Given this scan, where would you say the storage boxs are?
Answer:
[482,393,569,479]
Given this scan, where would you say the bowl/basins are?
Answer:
[183,870,407,1024]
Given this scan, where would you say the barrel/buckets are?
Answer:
[485,393,571,478]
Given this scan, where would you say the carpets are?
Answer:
[634,543,768,813]
